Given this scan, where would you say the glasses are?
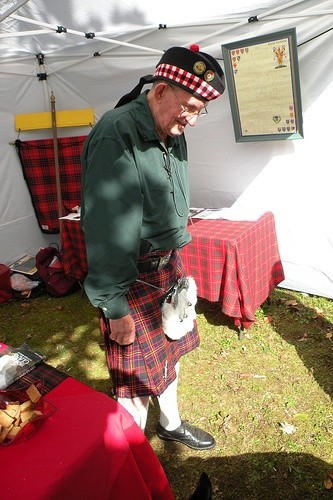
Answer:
[168,83,208,118]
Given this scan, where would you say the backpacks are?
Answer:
[35,246,77,297]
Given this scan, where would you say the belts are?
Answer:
[137,250,173,272]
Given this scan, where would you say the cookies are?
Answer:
[0,384,42,442]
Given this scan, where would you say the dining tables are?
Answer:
[58,206,285,340]
[0,341,175,500]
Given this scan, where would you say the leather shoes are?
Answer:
[156,421,216,450]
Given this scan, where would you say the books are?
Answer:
[0,343,45,390]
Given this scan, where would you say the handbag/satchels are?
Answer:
[162,277,198,340]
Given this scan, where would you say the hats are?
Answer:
[114,44,226,107]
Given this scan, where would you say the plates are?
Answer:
[0,380,57,446]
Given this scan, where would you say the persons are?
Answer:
[81,44,226,450]
